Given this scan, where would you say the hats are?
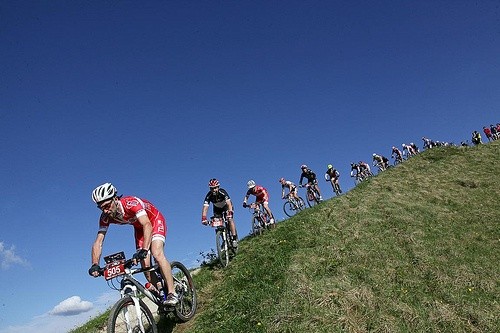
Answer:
[328,165,332,169]
[350,162,354,165]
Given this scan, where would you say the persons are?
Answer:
[401,144,416,155]
[461,142,468,146]
[472,123,500,145]
[410,142,419,153]
[358,160,372,176]
[243,180,274,227]
[372,153,389,171]
[390,146,403,161]
[88,183,179,305]
[325,164,342,192]
[422,137,456,149]
[350,161,364,180]
[202,178,238,248]
[298,165,323,200]
[279,178,303,209]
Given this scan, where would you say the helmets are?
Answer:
[92,183,117,203]
[301,165,307,169]
[208,179,220,187]
[247,180,256,188]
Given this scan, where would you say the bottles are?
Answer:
[156,280,164,296]
[145,282,159,297]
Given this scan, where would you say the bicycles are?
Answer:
[373,150,420,174]
[326,175,342,197]
[94,255,198,333]
[204,211,239,267]
[351,169,374,187]
[301,183,322,208]
[282,186,306,217]
[244,202,276,237]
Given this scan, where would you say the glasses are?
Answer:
[210,187,218,190]
[97,200,114,211]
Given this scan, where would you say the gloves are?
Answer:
[225,210,234,220]
[243,202,247,208]
[201,219,207,225]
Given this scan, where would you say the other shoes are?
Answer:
[232,240,238,248]
[319,196,324,201]
[339,189,342,193]
[270,218,274,224]
[300,203,303,207]
[163,292,179,307]
